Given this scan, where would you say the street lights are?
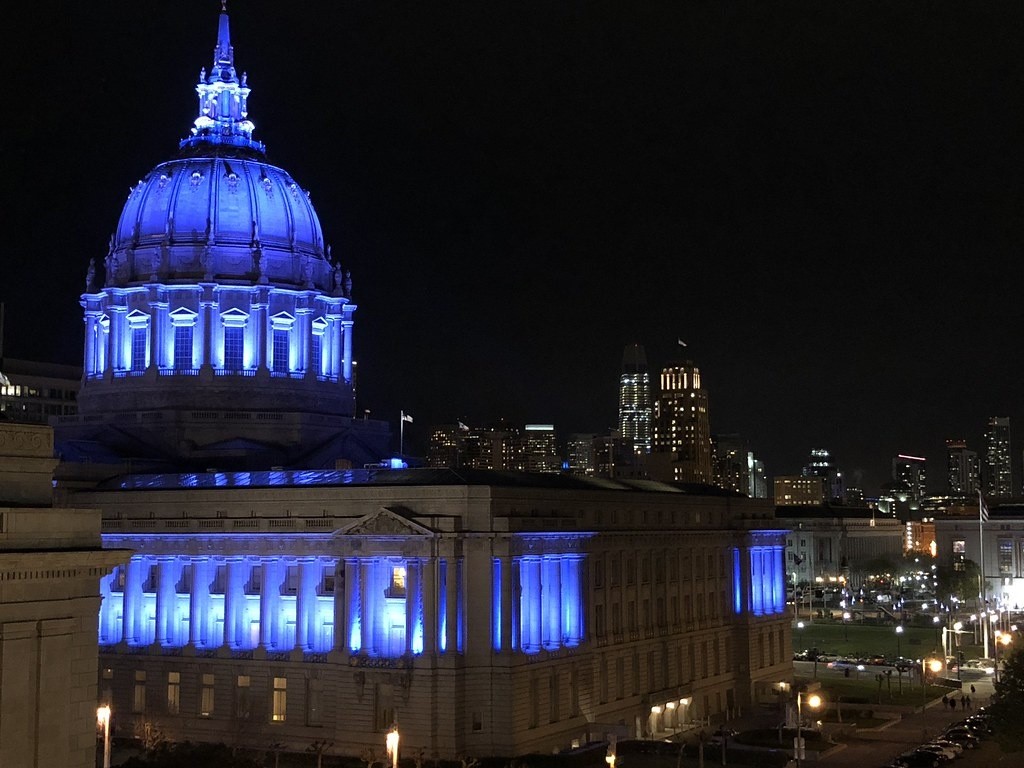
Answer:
[934,616,940,646]
[994,629,1000,681]
[953,621,962,647]
[797,621,804,654]
[970,614,978,645]
[896,625,903,658]
[843,612,850,643]
[999,607,1006,630]
[796,692,822,768]
[991,615,998,634]
[922,659,940,711]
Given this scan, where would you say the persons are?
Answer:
[966,694,972,711]
[942,693,949,711]
[970,683,976,698]
[950,695,957,712]
[961,696,965,712]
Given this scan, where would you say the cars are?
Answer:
[948,654,997,677]
[887,709,994,768]
[794,646,921,673]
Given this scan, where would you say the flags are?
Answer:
[980,493,990,523]
[793,554,802,567]
[402,411,414,423]
